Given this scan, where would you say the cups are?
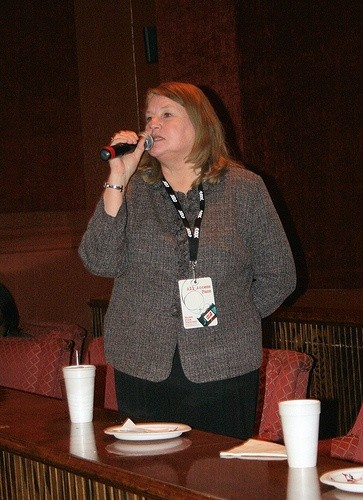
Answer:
[63,364,96,424]
[287,468,321,500]
[279,399,321,468]
[68,423,98,461]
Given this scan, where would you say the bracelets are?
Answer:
[102,181,127,193]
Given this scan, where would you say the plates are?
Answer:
[319,466,363,492]
[105,437,193,456]
[104,422,191,441]
[321,489,363,500]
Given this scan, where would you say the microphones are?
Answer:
[100,131,154,160]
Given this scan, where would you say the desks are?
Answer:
[0,384,363,500]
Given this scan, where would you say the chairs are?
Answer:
[317,400,363,465]
[0,334,74,399]
[21,317,86,365]
[81,334,117,410]
[257,347,314,442]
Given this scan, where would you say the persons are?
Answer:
[79,80,298,439]
